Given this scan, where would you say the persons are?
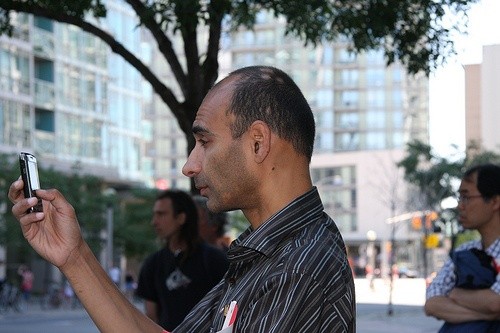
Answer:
[136,191,229,333]
[8,66,356,333]
[425,163,500,333]
[1,260,136,310]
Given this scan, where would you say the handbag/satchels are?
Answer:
[437,247,500,332]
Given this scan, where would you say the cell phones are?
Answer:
[19,152,44,214]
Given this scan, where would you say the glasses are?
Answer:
[457,195,482,202]
